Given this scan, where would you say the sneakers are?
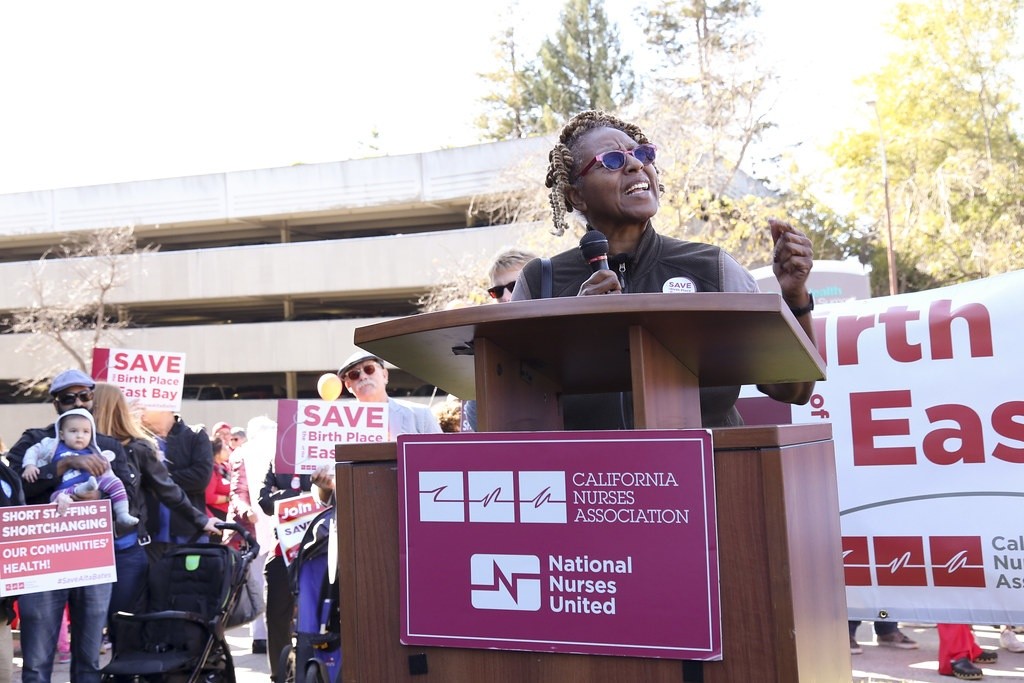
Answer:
[1001,629,1024,652]
[877,629,919,647]
[849,635,862,653]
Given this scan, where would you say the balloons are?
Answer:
[316,371,342,400]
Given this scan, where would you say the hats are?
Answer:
[49,370,96,395]
[211,422,231,436]
[54,408,101,452]
[337,352,385,382]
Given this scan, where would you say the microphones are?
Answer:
[579,230,613,295]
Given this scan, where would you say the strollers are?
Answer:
[270,504,342,683]
[91,521,259,683]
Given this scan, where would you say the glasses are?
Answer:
[54,389,95,405]
[487,280,516,298]
[231,435,245,442]
[347,363,382,380]
[575,143,657,180]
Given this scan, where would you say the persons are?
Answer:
[465,109,818,432]
[0,248,535,683]
[847,621,1024,680]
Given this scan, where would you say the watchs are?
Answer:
[792,290,815,317]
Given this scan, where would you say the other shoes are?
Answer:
[101,637,113,651]
[951,657,983,679]
[59,652,72,663]
[974,650,998,663]
[252,639,268,654]
